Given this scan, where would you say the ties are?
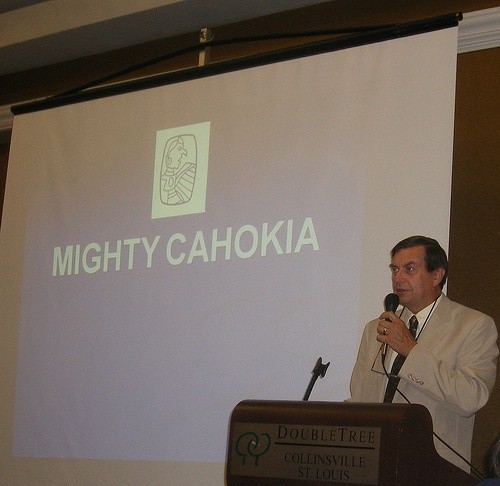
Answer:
[382,316,418,403]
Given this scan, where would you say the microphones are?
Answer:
[382,292,400,364]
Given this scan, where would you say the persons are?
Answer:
[349,236,498,474]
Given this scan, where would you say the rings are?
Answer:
[383,329,387,335]
